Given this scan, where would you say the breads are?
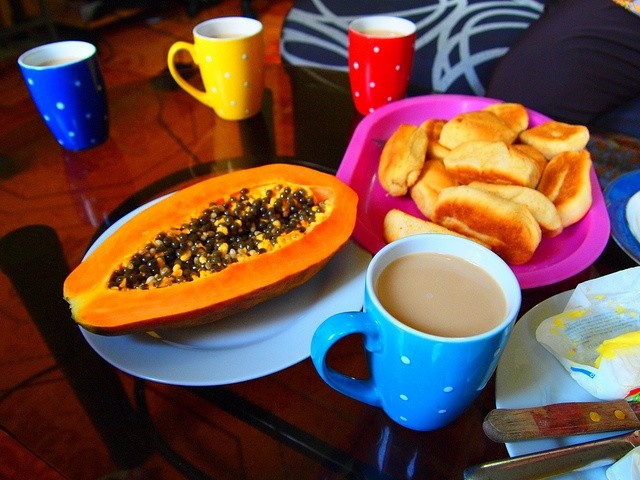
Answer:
[381,102,593,268]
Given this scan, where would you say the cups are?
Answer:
[167,16,265,122]
[17,41,109,151]
[348,14,415,117]
[311,235,522,431]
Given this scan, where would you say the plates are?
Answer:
[334,93,611,290]
[602,170,638,264]
[494,287,639,479]
[76,189,373,388]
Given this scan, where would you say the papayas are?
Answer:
[63,162,359,334]
